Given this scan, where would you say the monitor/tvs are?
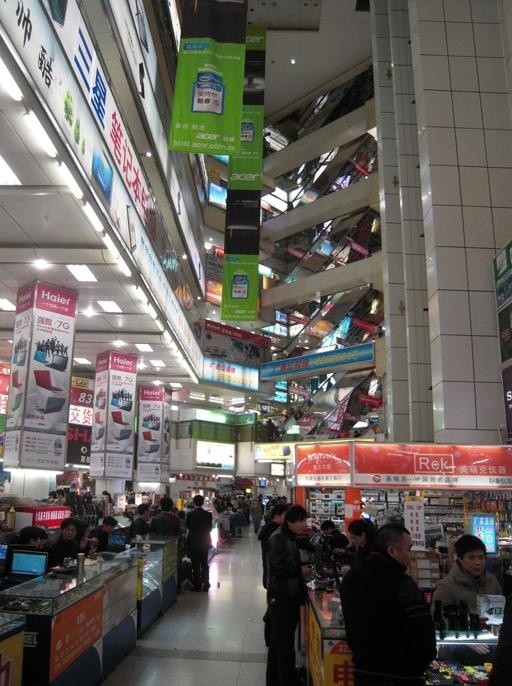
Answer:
[0,544,10,561]
[10,550,48,575]
[108,533,127,546]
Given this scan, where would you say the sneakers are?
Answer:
[190,586,209,592]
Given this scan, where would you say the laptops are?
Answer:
[49,0,67,26]
[10,351,68,413]
[90,149,113,214]
[95,396,170,454]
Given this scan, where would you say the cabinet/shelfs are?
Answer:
[0,496,195,686]
[297,488,510,685]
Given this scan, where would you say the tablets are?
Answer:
[126,205,137,253]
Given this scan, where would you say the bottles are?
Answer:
[76,552,86,575]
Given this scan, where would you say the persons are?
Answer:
[37,487,114,516]
[211,493,263,537]
[129,491,214,593]
[258,495,315,685]
[5,517,118,586]
[320,518,512,686]
